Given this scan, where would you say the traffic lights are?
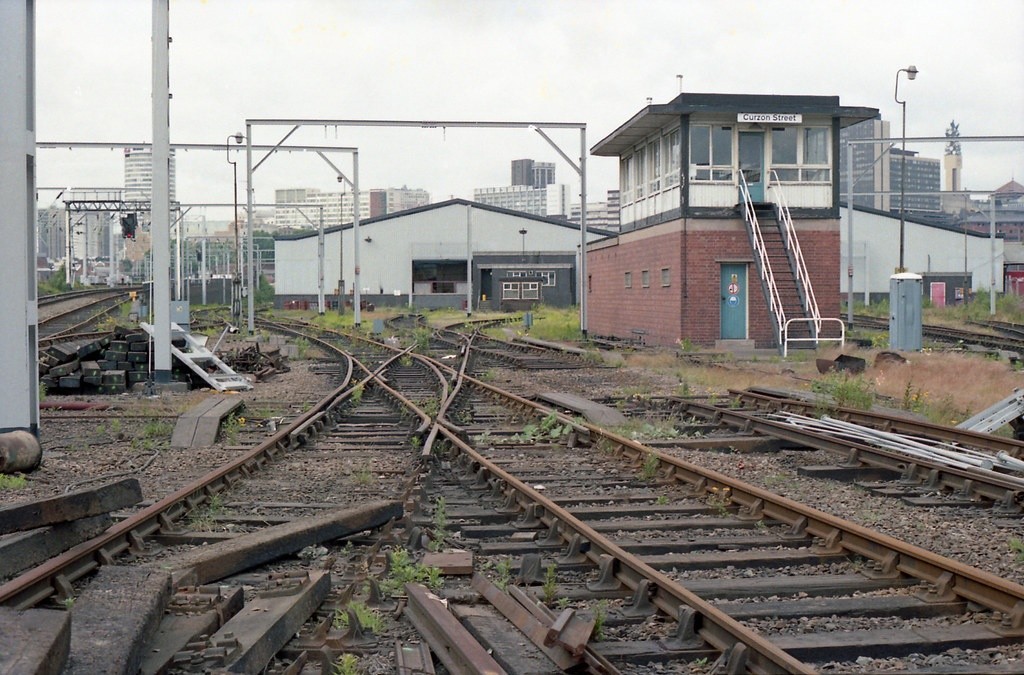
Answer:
[120,214,136,238]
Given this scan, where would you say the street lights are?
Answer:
[226,132,243,271]
[338,174,346,278]
[894,65,918,272]
